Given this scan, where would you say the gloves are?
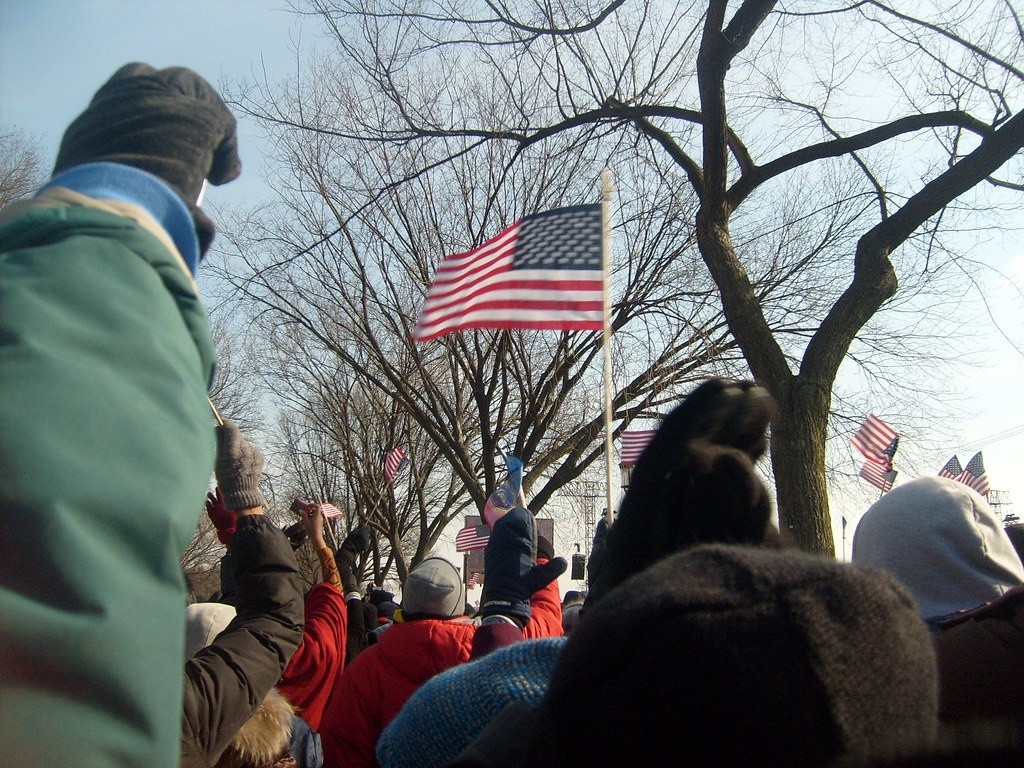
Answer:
[340,525,370,561]
[50,60,242,262]
[213,421,264,509]
[583,382,783,618]
[344,574,359,596]
[472,508,568,625]
[206,488,238,543]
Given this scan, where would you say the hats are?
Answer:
[403,556,465,616]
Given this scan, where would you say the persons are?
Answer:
[181,378,1024,767]
[0,59,251,768]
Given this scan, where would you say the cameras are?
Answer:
[289,498,313,518]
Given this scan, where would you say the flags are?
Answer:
[619,429,659,468]
[410,202,606,340]
[858,459,898,494]
[956,450,991,497]
[318,503,345,520]
[851,413,901,469]
[937,455,961,480]
[384,446,410,485]
[455,523,491,553]
